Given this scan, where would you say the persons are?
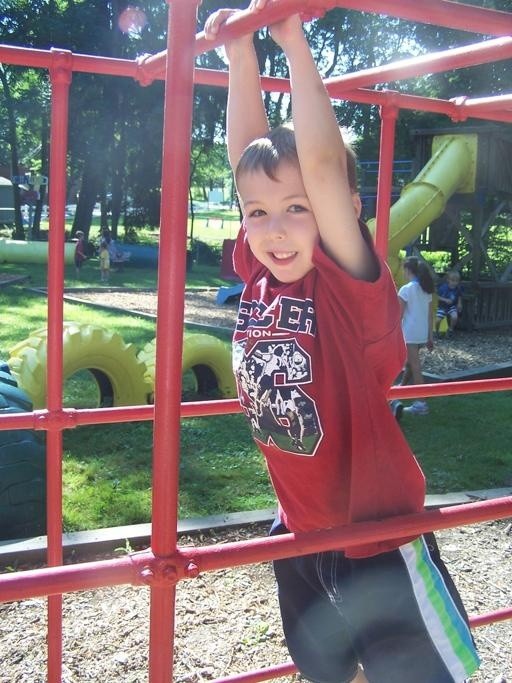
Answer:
[73,225,124,283]
[396,255,436,416]
[387,399,404,424]
[431,268,464,341]
[202,0,484,683]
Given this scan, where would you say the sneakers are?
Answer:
[432,329,454,340]
[402,400,430,416]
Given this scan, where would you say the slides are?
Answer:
[367,132,477,336]
